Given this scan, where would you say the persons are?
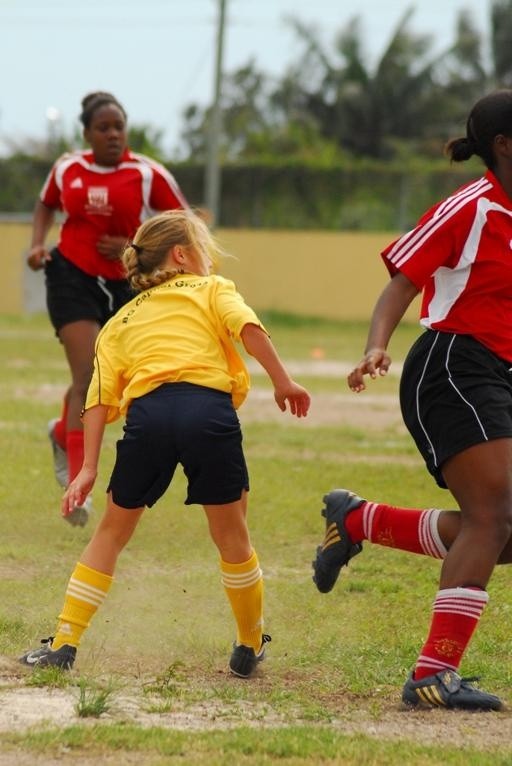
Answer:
[9,202,315,678]
[25,90,198,527]
[306,87,512,712]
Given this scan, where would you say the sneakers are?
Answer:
[229,640,266,678]
[313,488,368,593]
[47,419,69,491]
[61,488,93,529]
[402,667,503,711]
[16,637,78,673]
